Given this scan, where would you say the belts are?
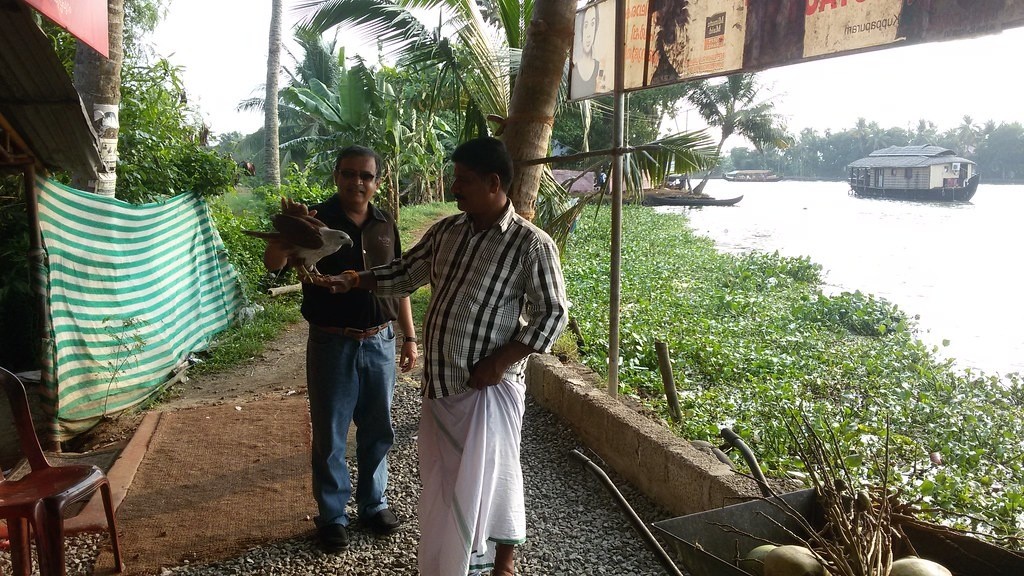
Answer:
[311,322,390,339]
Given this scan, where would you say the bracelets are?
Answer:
[403,336,417,343]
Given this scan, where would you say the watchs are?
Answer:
[342,270,360,287]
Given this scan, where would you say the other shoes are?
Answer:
[359,507,400,529]
[320,523,350,552]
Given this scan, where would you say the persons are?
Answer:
[264,146,418,551]
[571,4,606,99]
[296,136,569,576]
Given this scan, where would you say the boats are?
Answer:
[725,170,779,182]
[847,143,980,202]
[641,172,743,207]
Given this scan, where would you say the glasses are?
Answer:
[336,170,377,181]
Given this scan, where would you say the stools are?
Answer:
[1,367,126,575]
